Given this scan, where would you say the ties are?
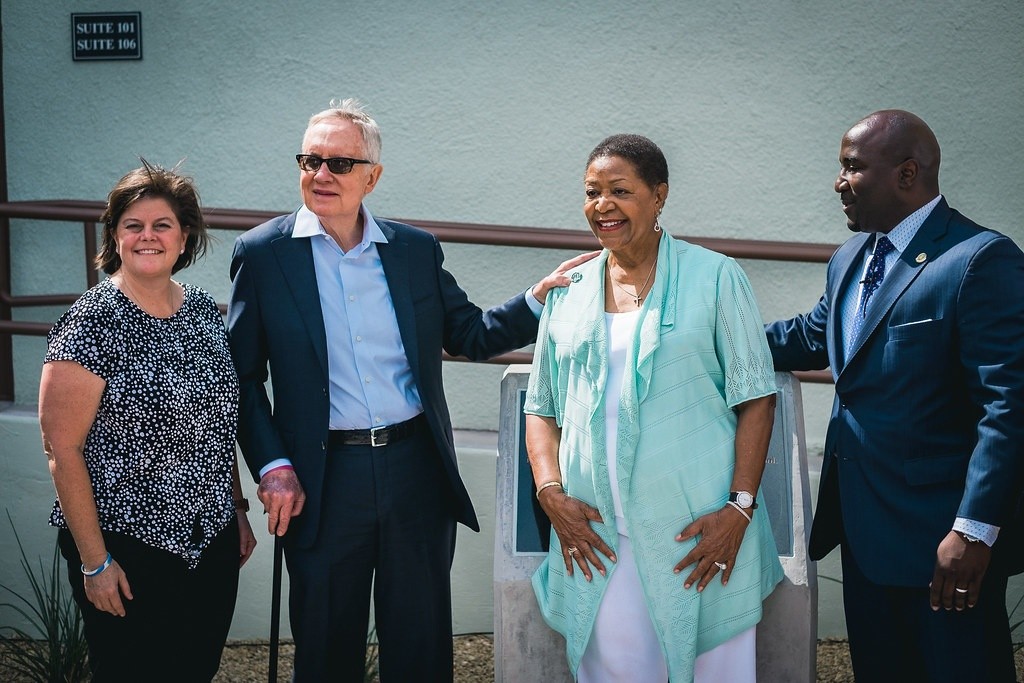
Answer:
[846,236,898,371]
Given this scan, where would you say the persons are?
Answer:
[754,105,1024,683]
[225,96,605,683]
[37,159,257,683]
[520,134,785,683]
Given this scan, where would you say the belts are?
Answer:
[328,414,428,448]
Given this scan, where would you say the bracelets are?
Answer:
[262,465,295,477]
[80,553,112,577]
[536,482,561,501]
[232,498,249,512]
[726,500,752,523]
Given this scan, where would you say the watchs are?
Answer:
[962,533,981,543]
[728,489,758,510]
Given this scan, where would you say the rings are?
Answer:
[714,561,727,570]
[955,588,968,593]
[567,547,579,556]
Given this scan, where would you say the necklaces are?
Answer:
[117,264,175,320]
[606,244,665,306]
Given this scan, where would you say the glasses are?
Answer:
[295,154,373,175]
